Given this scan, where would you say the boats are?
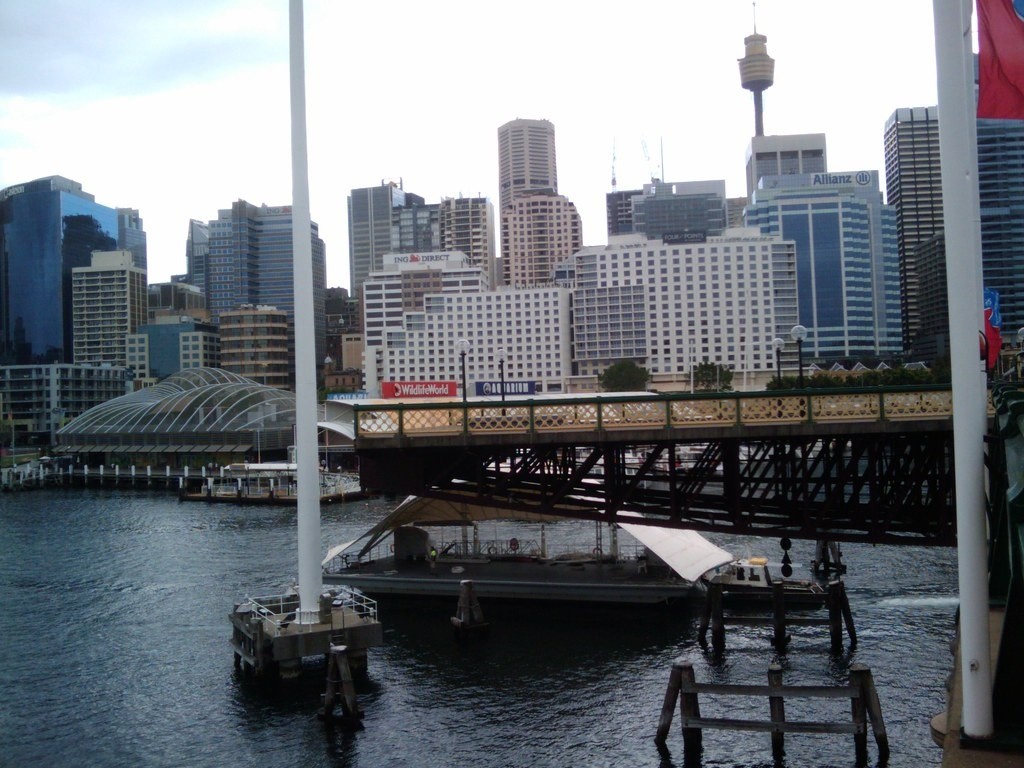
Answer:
[322,463,829,616]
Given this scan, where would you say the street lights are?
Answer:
[455,339,470,431]
[791,325,807,387]
[772,338,785,389]
[495,349,507,416]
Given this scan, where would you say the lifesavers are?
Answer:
[488,546,497,554]
[593,548,602,555]
[509,539,519,550]
[749,557,767,565]
[390,544,394,553]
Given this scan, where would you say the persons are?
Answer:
[321,458,326,468]
[430,546,437,575]
